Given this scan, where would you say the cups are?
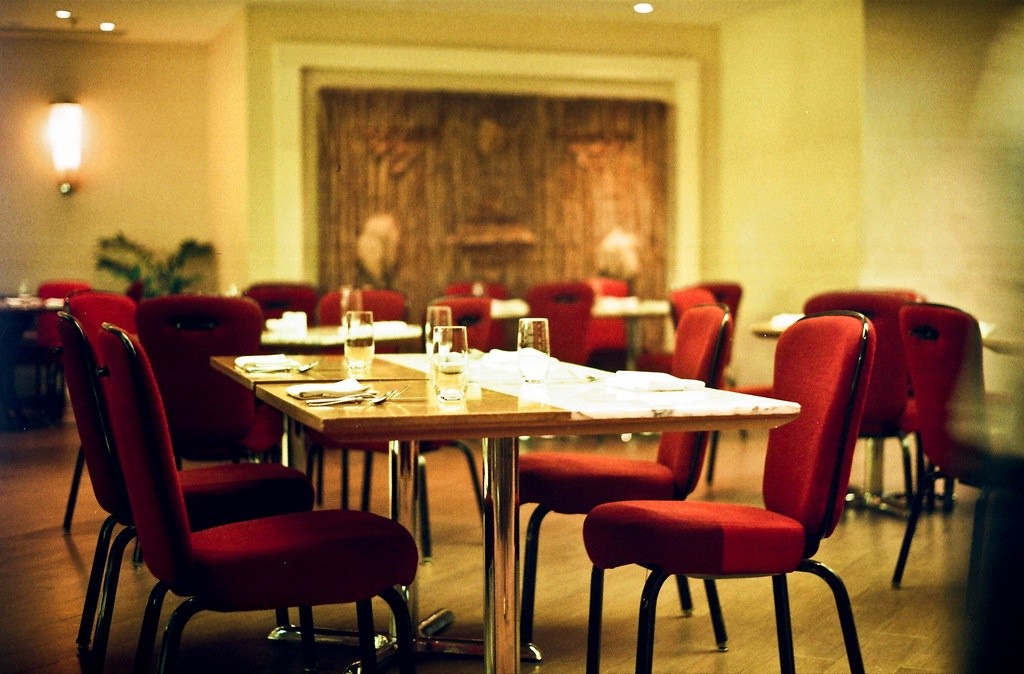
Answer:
[342,309,375,380]
[339,285,364,325]
[432,326,468,402]
[517,318,550,383]
[17,278,32,309]
[425,307,452,371]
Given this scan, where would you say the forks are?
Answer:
[309,390,397,407]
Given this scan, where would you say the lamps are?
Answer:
[47,97,84,195]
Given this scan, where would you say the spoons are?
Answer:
[249,360,319,372]
[306,396,388,404]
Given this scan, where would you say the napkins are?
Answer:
[234,311,808,420]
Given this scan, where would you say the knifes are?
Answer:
[298,387,369,398]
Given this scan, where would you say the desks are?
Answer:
[260,321,422,351]
[213,351,545,674]
[0,296,66,433]
[255,372,803,674]
[591,298,670,440]
[490,300,562,445]
[751,322,998,522]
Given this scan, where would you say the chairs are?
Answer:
[316,291,406,352]
[582,308,881,674]
[61,290,138,534]
[38,279,92,415]
[579,278,632,372]
[637,290,717,374]
[429,297,505,350]
[524,282,597,368]
[519,304,736,665]
[892,301,989,591]
[699,281,750,445]
[56,311,315,674]
[448,282,509,351]
[804,288,917,519]
[243,283,319,327]
[96,321,420,674]
[131,295,298,469]
